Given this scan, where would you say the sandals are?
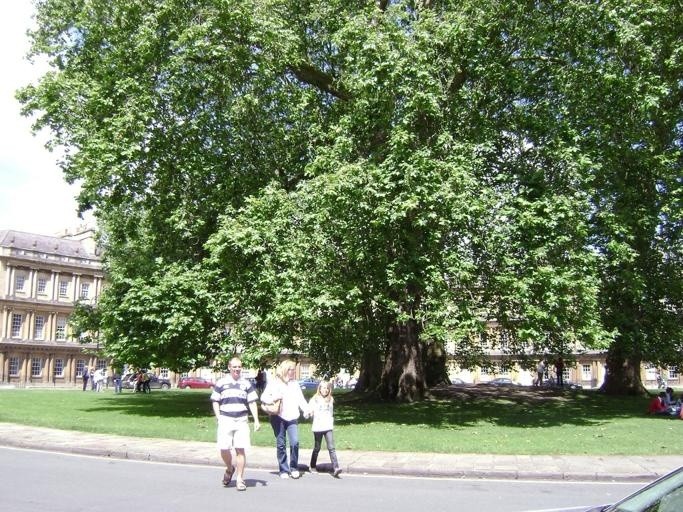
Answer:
[236,479,247,491]
[223,466,236,485]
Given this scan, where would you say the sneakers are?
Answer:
[309,465,319,474]
[290,471,300,479]
[281,472,289,478]
[333,468,342,476]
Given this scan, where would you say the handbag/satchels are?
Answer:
[260,399,281,416]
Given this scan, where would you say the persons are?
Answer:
[81,365,152,394]
[209,357,262,492]
[257,358,313,482]
[302,379,342,479]
[532,359,546,387]
[552,357,564,387]
[647,386,682,421]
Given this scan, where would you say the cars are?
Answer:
[104,368,358,391]
[448,376,585,390]
[520,462,683,511]
